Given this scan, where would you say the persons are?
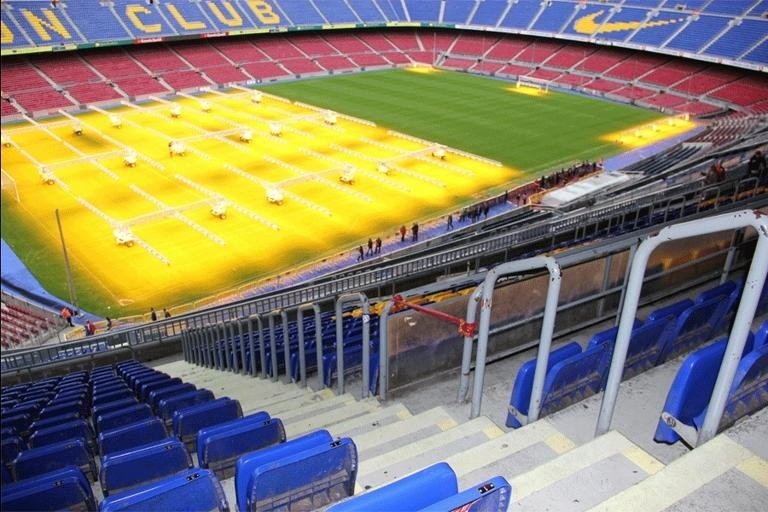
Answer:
[62,307,74,327]
[516,192,527,206]
[411,222,418,241]
[541,159,596,189]
[150,308,156,321]
[358,237,381,261]
[163,309,171,319]
[447,212,454,230]
[106,316,112,331]
[400,225,406,241]
[85,319,95,336]
[705,151,768,185]
[459,204,489,223]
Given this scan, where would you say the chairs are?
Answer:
[0,278,768,512]
